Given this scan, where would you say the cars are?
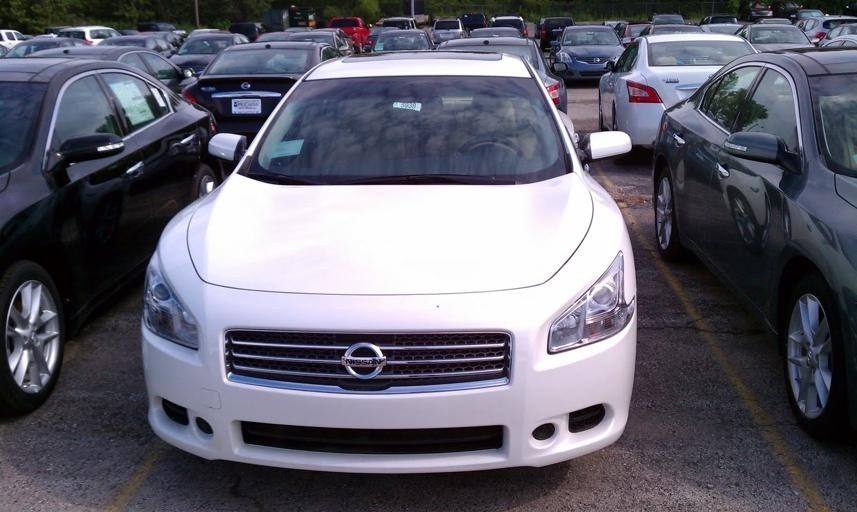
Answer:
[0,48,228,425]
[779,192,857,338]
[138,44,637,478]
[651,46,857,439]
[714,164,772,252]
